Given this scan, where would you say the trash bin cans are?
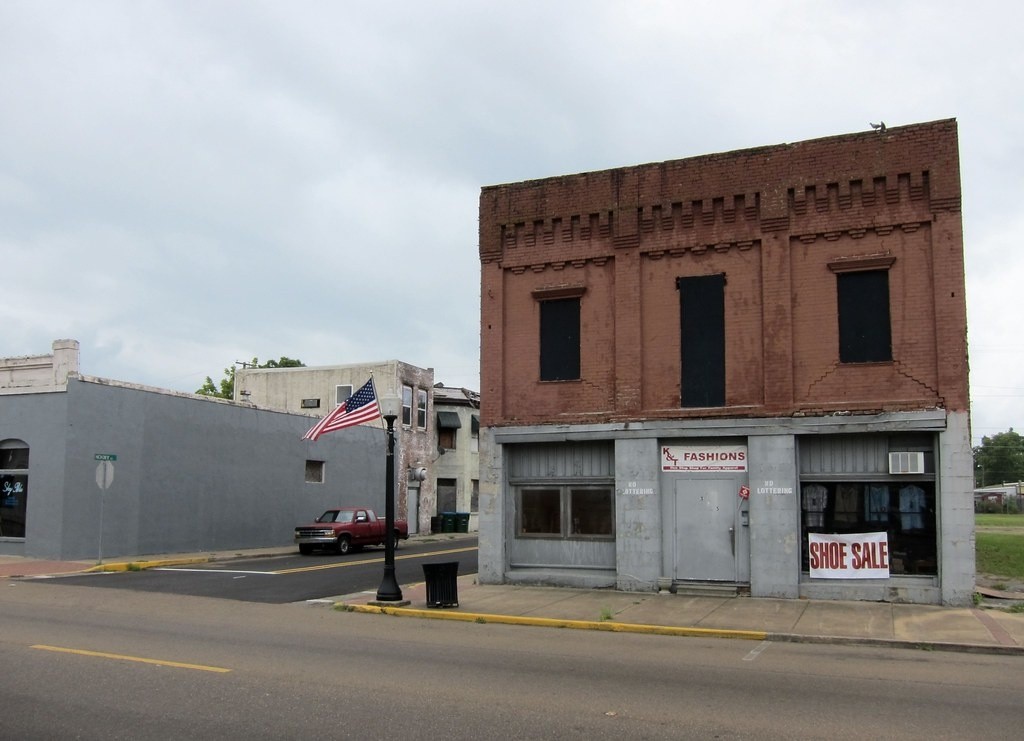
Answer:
[431,514,444,533]
[455,512,471,533]
[437,511,457,533]
[420,561,460,609]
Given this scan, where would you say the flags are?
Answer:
[300,376,381,442]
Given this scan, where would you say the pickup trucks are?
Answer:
[294,506,409,556]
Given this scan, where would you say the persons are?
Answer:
[1002,491,1008,514]
